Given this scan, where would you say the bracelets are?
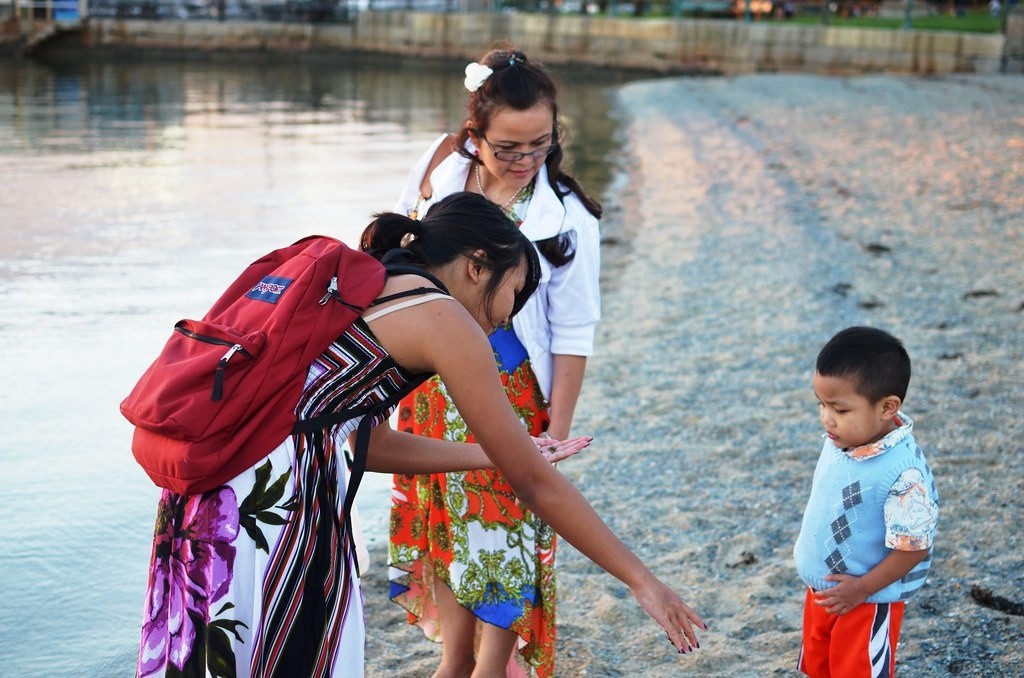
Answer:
[538,432,550,440]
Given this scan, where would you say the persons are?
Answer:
[793,327,939,678]
[134,193,707,678]
[388,46,603,678]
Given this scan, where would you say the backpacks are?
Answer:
[120,236,449,519]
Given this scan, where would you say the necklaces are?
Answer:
[475,162,521,209]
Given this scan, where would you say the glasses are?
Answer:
[482,134,556,162]
